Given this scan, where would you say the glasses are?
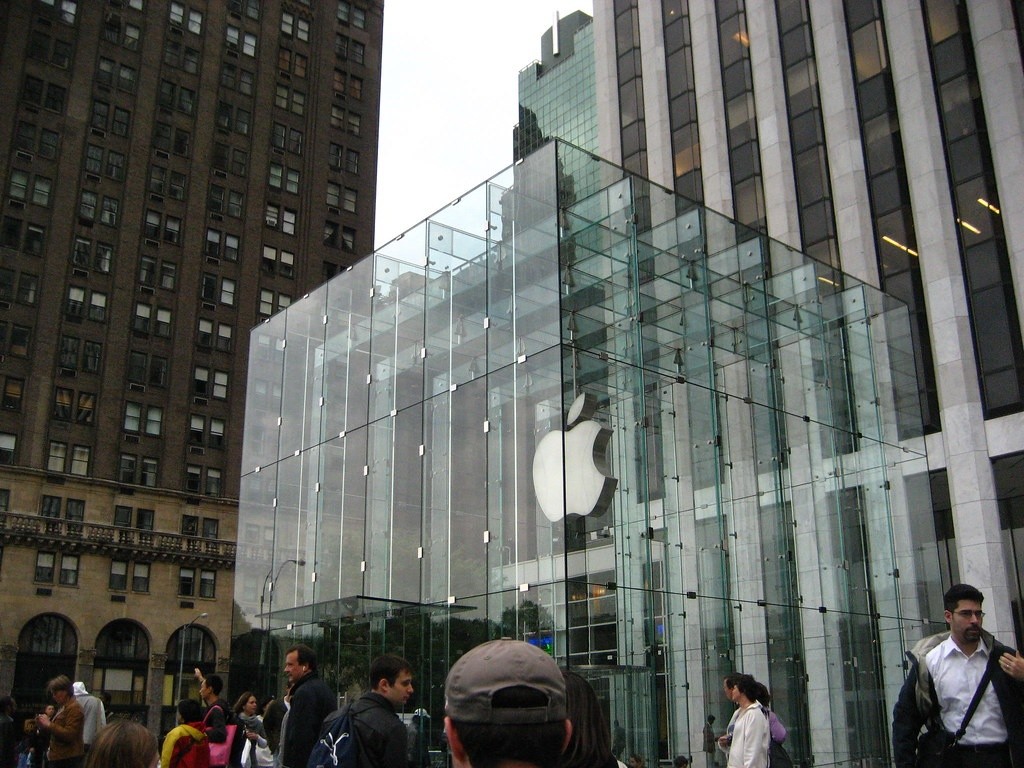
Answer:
[952,610,985,619]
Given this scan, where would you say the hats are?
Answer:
[413,708,431,719]
[444,637,567,723]
[72,682,89,696]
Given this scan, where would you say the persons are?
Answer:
[407,708,452,768]
[320,653,414,768]
[628,754,647,768]
[703,715,719,768]
[612,719,626,762]
[672,755,688,768]
[561,670,628,768]
[892,584,1024,768]
[278,644,338,768]
[717,672,787,768]
[0,668,295,768]
[442,637,573,768]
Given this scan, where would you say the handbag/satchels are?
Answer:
[916,730,962,768]
[768,710,789,744]
[203,705,237,766]
[767,732,793,768]
[240,725,253,768]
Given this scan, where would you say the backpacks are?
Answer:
[307,702,380,768]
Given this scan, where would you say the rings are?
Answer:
[1007,664,1009,667]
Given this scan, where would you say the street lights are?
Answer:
[269,559,306,612]
[175,613,208,727]
